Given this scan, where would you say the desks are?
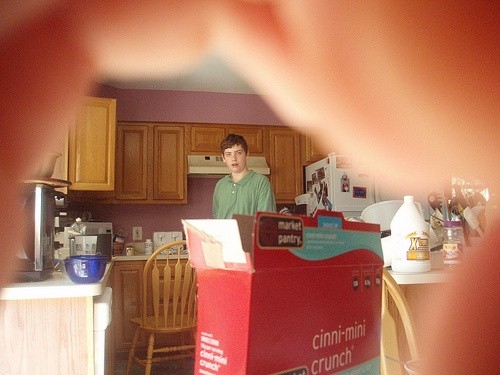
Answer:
[386,267,455,310]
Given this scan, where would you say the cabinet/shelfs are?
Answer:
[117,120,189,205]
[266,125,304,205]
[302,126,328,166]
[113,260,197,360]
[67,94,117,189]
[74,120,117,206]
[188,122,267,157]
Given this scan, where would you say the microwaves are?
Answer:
[69,222,114,260]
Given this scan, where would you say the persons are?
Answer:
[212,132,277,220]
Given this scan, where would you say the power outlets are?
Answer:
[131,226,143,241]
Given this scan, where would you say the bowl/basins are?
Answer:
[64,255,107,284]
[361,200,403,229]
[20,148,62,177]
[113,242,124,255]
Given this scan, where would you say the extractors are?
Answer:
[187,154,271,178]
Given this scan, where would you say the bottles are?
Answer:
[389,195,433,273]
[442,220,466,273]
[125,246,134,256]
[145,239,153,255]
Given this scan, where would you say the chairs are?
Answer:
[360,199,426,231]
[381,266,419,375]
[124,239,198,374]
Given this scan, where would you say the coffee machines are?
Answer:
[10,183,57,282]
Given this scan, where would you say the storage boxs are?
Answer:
[181,209,385,375]
[125,246,136,256]
[68,234,111,262]
[113,241,126,257]
[152,230,183,255]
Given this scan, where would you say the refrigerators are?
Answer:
[305,154,375,219]
